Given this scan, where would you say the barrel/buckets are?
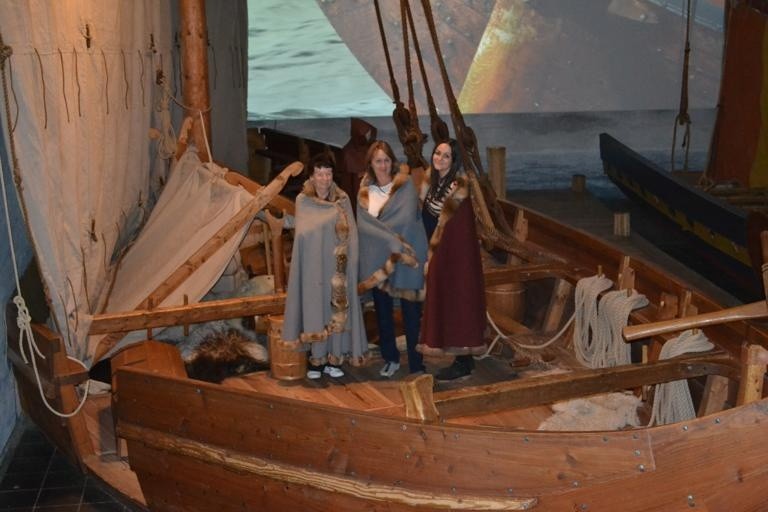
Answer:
[483,280,527,333]
[267,315,308,381]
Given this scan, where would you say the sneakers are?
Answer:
[307,371,322,379]
[435,357,475,382]
[324,366,344,378]
[380,361,401,376]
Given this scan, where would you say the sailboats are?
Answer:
[0,0,768,511]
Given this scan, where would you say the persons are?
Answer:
[415,139,489,380]
[355,139,428,380]
[279,149,369,382]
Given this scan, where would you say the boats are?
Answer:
[576,2,767,299]
